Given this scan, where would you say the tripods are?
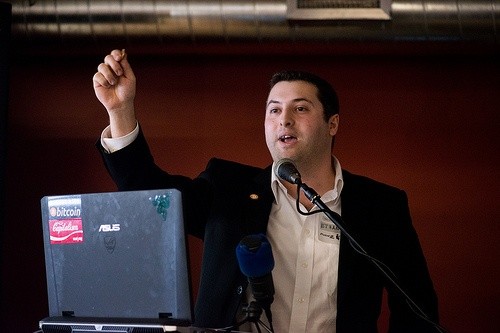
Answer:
[221,301,273,333]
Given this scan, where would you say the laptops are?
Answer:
[39,189,228,333]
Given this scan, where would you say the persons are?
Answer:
[93,49,440,333]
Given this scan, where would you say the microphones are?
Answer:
[236,235,275,323]
[274,158,301,184]
